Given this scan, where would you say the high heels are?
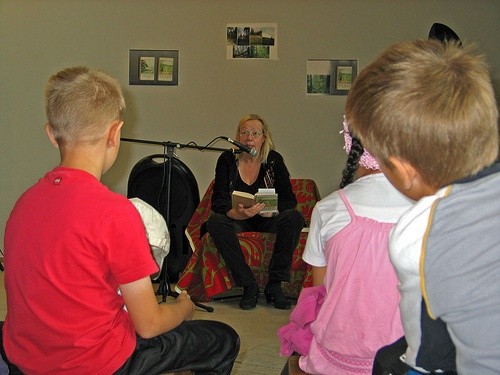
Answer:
[240,288,260,310]
[264,281,291,310]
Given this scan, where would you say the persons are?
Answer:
[0,66,243,374]
[209,115,303,310]
[282,38,500,375]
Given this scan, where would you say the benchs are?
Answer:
[173,179,321,303]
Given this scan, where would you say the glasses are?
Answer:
[240,131,262,138]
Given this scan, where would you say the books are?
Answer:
[231,190,278,211]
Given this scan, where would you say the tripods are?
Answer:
[119,138,242,312]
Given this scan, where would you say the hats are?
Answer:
[128,197,170,281]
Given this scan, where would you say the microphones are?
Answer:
[220,136,258,157]
